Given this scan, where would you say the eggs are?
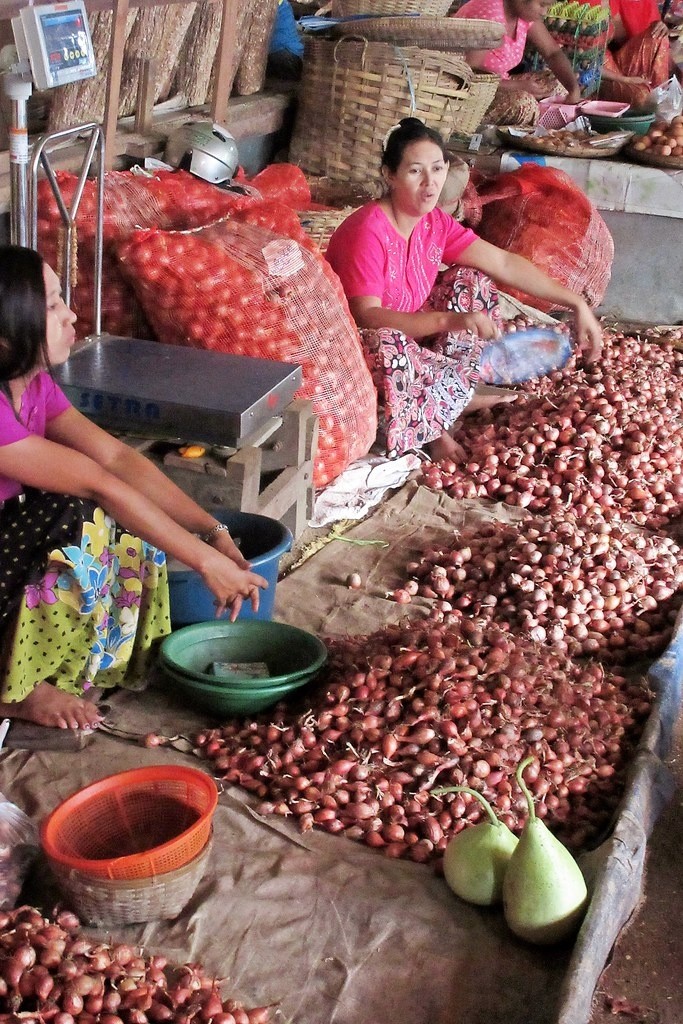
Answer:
[543,17,603,82]
[631,115,683,158]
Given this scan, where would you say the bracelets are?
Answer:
[202,524,228,543]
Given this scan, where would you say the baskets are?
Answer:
[626,143,683,171]
[452,64,500,138]
[496,123,626,158]
[48,786,215,927]
[332,0,453,23]
[287,34,474,197]
[39,764,219,878]
[334,16,507,53]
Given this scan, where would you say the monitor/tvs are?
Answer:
[12,0,98,92]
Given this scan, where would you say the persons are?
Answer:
[554,0,683,124]
[449,0,582,131]
[323,115,604,465]
[0,240,272,731]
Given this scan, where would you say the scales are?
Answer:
[4,1,303,440]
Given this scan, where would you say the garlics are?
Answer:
[138,311,681,869]
[1,902,284,1023]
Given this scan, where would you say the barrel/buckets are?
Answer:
[157,509,295,630]
